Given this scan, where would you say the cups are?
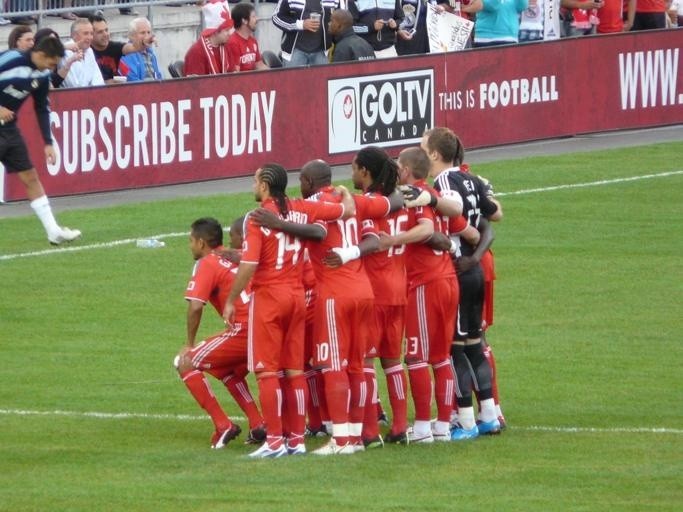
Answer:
[309,13,322,32]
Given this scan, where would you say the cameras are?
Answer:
[383,21,389,27]
[408,28,416,36]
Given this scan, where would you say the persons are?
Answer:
[0,38,82,247]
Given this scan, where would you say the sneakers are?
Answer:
[362,433,385,450]
[430,429,452,442]
[406,428,434,444]
[497,415,506,429]
[475,419,501,435]
[382,428,410,449]
[377,411,391,427]
[348,438,365,453]
[199,0,235,37]
[309,437,355,456]
[449,421,481,441]
[244,423,268,446]
[0,5,142,28]
[210,420,242,450]
[303,423,328,438]
[284,441,306,456]
[247,439,289,461]
[47,226,82,247]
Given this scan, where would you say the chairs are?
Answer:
[167,49,282,79]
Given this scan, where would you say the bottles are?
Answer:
[136,239,166,248]
